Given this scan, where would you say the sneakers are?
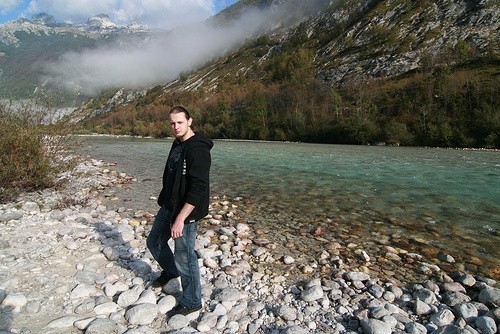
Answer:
[169,303,202,318]
[153,269,178,287]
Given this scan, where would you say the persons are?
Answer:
[146,104,216,321]
